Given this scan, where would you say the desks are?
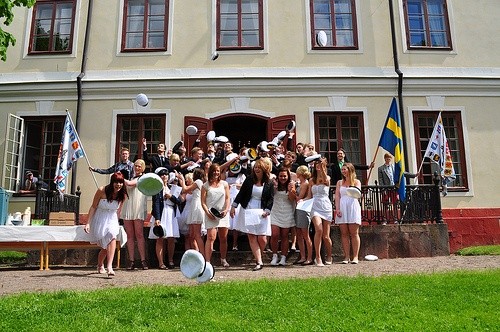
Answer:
[0,225,128,271]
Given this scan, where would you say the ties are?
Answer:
[340,162,342,168]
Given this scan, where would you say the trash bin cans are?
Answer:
[0,187,10,225]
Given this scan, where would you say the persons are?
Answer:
[377,153,420,225]
[84,129,375,280]
[19,171,38,194]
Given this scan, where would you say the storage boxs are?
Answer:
[49,211,76,226]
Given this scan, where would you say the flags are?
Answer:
[425,113,456,196]
[53,114,85,201]
[379,97,405,201]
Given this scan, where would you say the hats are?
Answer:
[211,52,220,61]
[155,167,168,174]
[180,249,206,279]
[230,163,241,173]
[214,136,229,144]
[226,153,238,162]
[26,171,32,176]
[136,173,163,197]
[258,120,322,163]
[246,148,256,160]
[186,125,198,136]
[206,131,215,141]
[316,30,328,47]
[136,93,148,108]
[195,261,215,282]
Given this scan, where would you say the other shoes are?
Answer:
[97,265,105,273]
[383,221,387,226]
[395,220,399,224]
[107,267,116,276]
[127,256,359,270]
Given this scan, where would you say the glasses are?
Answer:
[159,172,170,176]
